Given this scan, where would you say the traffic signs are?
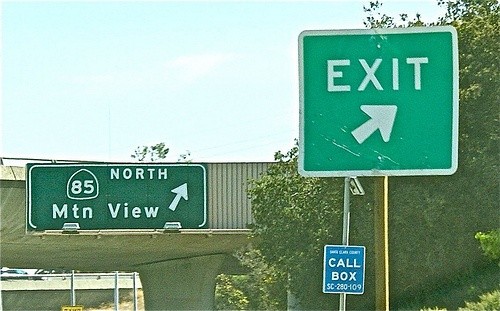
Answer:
[20,158,209,235]
[296,25,461,180]
